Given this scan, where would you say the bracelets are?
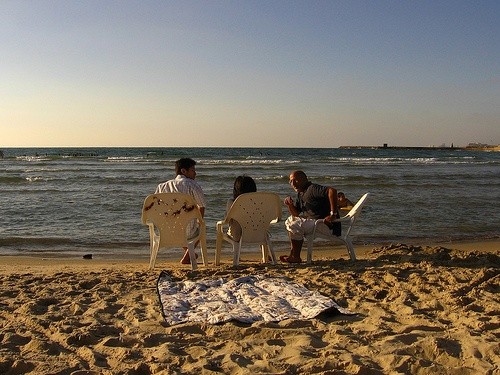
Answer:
[329,211,338,216]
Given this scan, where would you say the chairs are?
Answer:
[302,193,370,265]
[215,191,283,267]
[141,191,209,270]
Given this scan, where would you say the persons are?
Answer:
[336,192,353,216]
[227,175,278,263]
[153,158,205,264]
[279,171,342,263]
